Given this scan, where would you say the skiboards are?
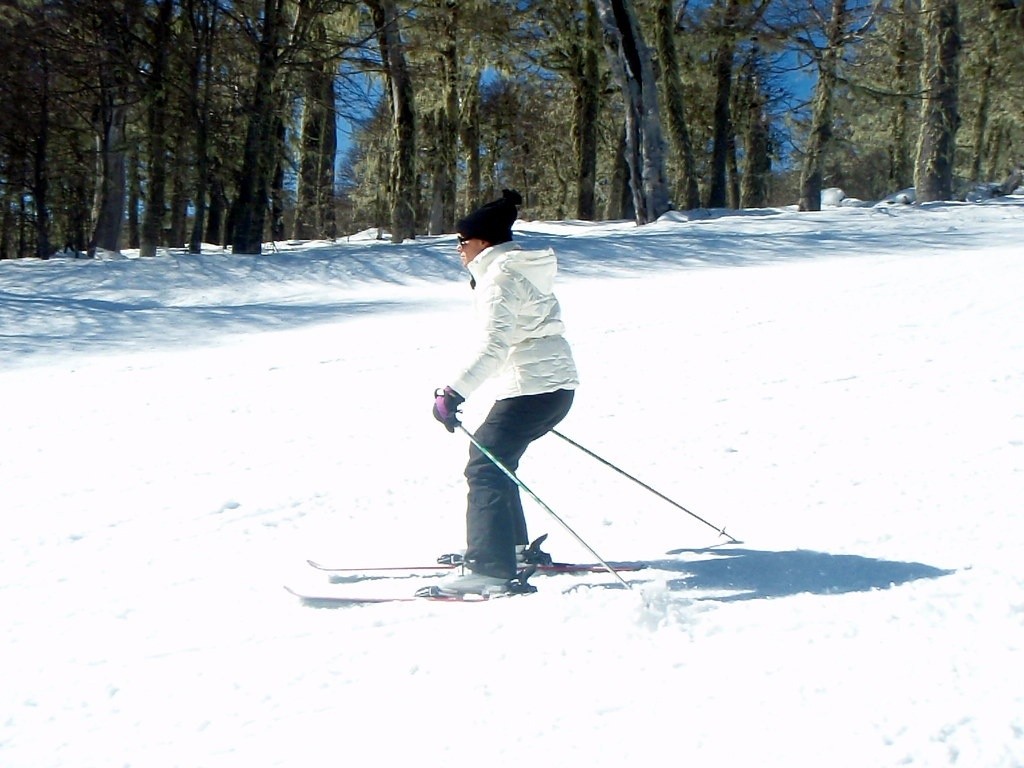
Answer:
[283,559,641,609]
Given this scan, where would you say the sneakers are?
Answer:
[440,545,528,595]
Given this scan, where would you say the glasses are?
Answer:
[457,235,470,246]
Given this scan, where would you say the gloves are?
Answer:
[432,387,465,433]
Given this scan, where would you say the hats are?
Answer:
[456,189,522,243]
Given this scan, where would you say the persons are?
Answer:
[414,189,579,597]
